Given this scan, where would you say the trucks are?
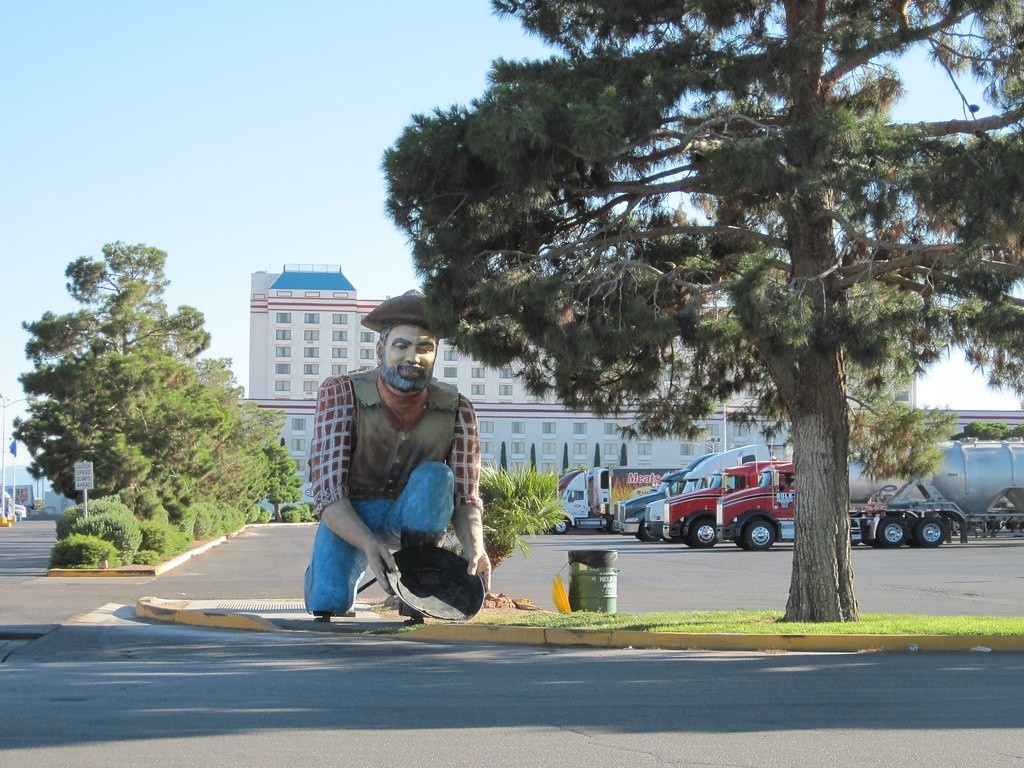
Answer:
[0,483,35,523]
[545,465,687,536]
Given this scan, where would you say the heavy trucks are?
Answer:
[716,437,1024,551]
[612,443,794,552]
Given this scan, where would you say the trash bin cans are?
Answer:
[567,550,618,614]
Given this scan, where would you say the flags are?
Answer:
[9,440,16,457]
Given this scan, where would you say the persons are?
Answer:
[303,290,492,610]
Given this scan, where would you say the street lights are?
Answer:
[0,392,49,528]
